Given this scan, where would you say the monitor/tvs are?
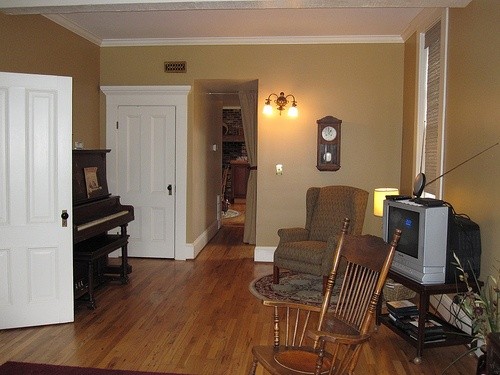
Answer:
[382,200,481,286]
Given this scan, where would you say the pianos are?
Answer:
[72,148,135,310]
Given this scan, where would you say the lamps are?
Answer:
[262,91,299,119]
[373,187,399,217]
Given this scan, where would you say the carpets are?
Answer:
[249,271,387,313]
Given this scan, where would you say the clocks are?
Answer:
[315,116,343,172]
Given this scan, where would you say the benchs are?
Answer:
[77,233,131,310]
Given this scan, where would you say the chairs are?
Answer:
[247,215,403,375]
[273,184,368,293]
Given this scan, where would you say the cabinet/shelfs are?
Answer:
[230,164,249,201]
[374,268,485,365]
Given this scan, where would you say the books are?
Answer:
[386,300,446,344]
